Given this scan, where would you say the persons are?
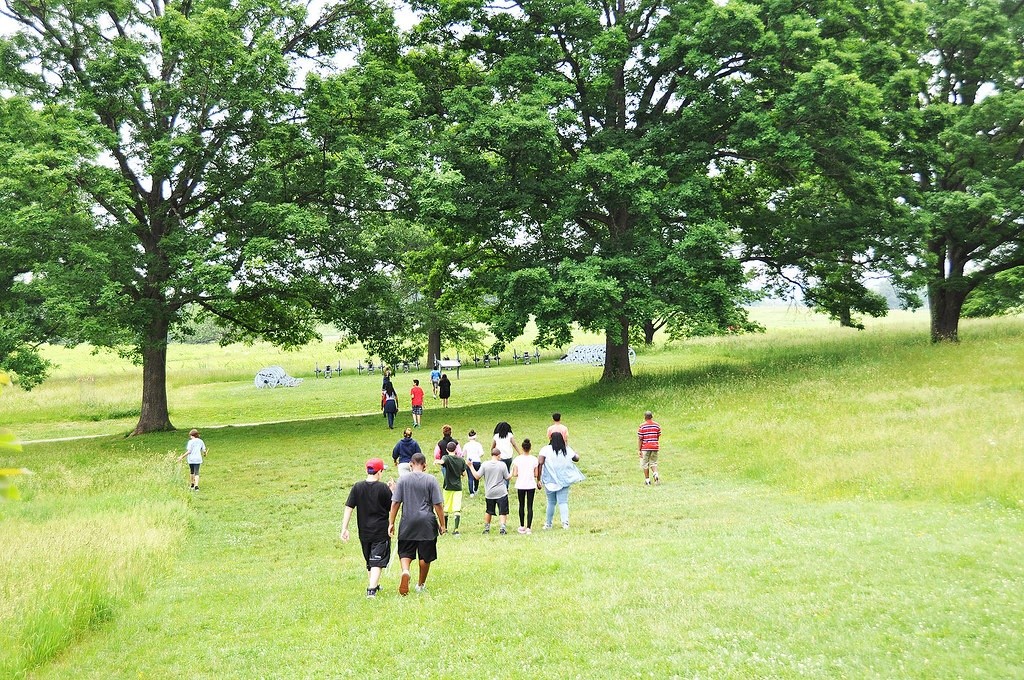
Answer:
[429,366,442,400]
[388,452,446,597]
[638,411,661,485]
[410,380,424,428]
[392,428,421,477]
[339,459,395,598]
[434,413,587,536]
[380,367,399,429]
[438,374,451,408]
[179,428,207,491]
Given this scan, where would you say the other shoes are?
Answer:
[367,584,383,598]
[398,570,411,596]
[413,422,421,428]
[190,483,199,492]
[517,526,532,535]
[654,476,659,482]
[500,529,507,534]
[470,488,480,498]
[452,531,459,535]
[415,583,426,591]
[481,526,490,534]
[646,482,651,485]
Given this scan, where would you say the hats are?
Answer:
[365,458,389,474]
